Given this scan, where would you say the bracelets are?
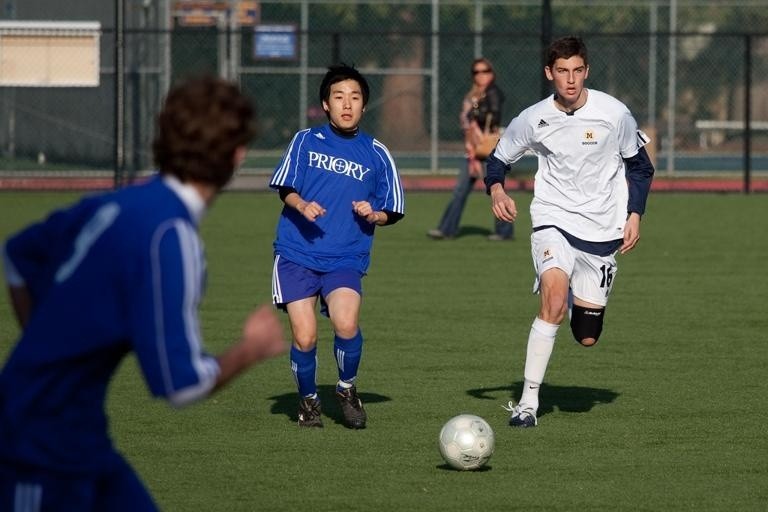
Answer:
[369,213,380,225]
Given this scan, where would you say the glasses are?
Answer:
[473,69,491,75]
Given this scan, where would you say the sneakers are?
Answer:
[334,383,366,428]
[488,234,513,241]
[298,397,324,429]
[500,401,537,428]
[428,230,454,239]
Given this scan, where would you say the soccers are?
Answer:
[437,414,495,470]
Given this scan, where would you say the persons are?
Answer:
[0,74,292,511]
[267,60,407,428]
[483,34,656,428]
[423,57,516,242]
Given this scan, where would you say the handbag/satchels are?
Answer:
[475,136,500,159]
